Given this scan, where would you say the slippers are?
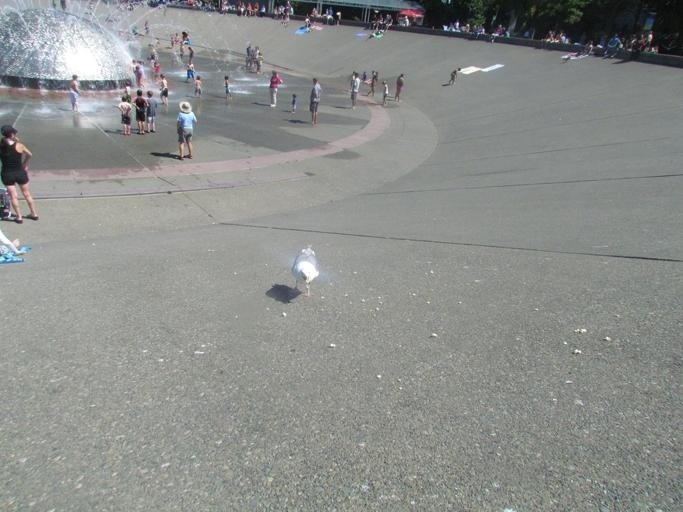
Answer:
[15,217,23,223]
[27,215,38,220]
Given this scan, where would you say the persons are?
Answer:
[371,10,393,35]
[176,102,197,160]
[0,125,39,224]
[291,94,297,114]
[246,43,263,74]
[449,68,461,85]
[224,76,232,100]
[269,70,282,108]
[0,229,26,255]
[118,80,158,135]
[117,1,203,107]
[351,71,404,109]
[310,78,321,125]
[305,7,341,30]
[543,30,653,60]
[403,16,409,27]
[202,1,293,27]
[453,20,509,44]
[70,74,81,113]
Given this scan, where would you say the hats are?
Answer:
[179,101,191,112]
[2,125,17,136]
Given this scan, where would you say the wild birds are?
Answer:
[291,245,320,297]
